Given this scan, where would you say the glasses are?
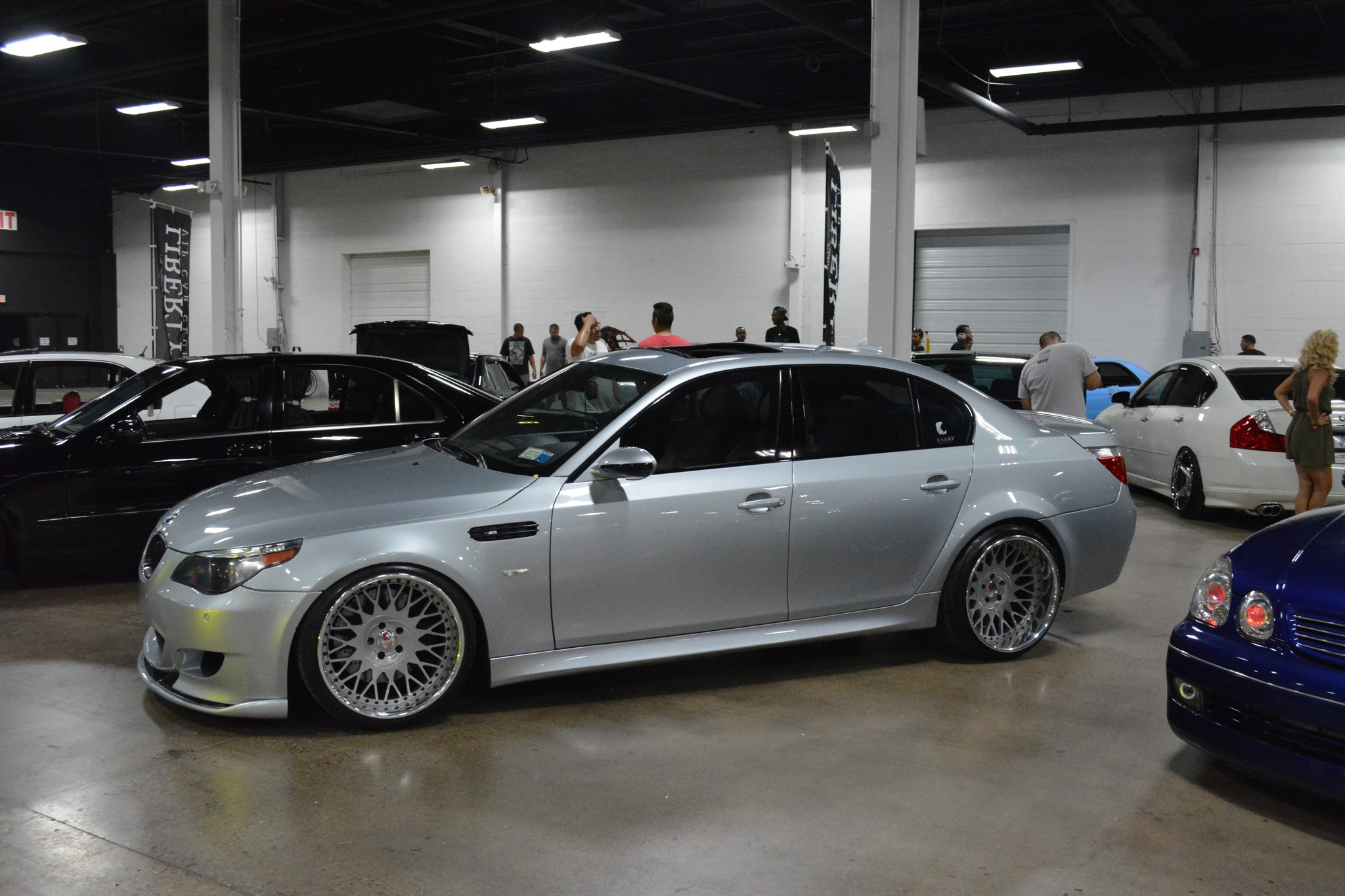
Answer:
[591,322,601,331]
[738,332,746,336]
[913,328,921,331]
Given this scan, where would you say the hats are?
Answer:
[774,306,788,320]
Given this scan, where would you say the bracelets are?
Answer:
[541,370,543,371]
[1289,410,1293,415]
[533,369,536,371]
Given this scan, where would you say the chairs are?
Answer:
[48,390,80,413]
[187,391,260,436]
[10,369,36,415]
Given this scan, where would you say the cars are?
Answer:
[1085,358,1153,422]
[1167,506,1345,806]
[0,350,608,582]
[908,351,1036,416]
[1091,355,1345,520]
[139,340,1139,734]
[0,352,211,436]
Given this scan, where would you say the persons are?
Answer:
[765,306,800,343]
[912,328,925,351]
[500,323,537,385]
[565,311,629,420]
[733,326,746,342]
[950,324,973,385]
[1017,331,1101,419]
[636,302,690,397]
[1237,334,1266,355]
[540,324,568,410]
[1274,330,1340,515]
